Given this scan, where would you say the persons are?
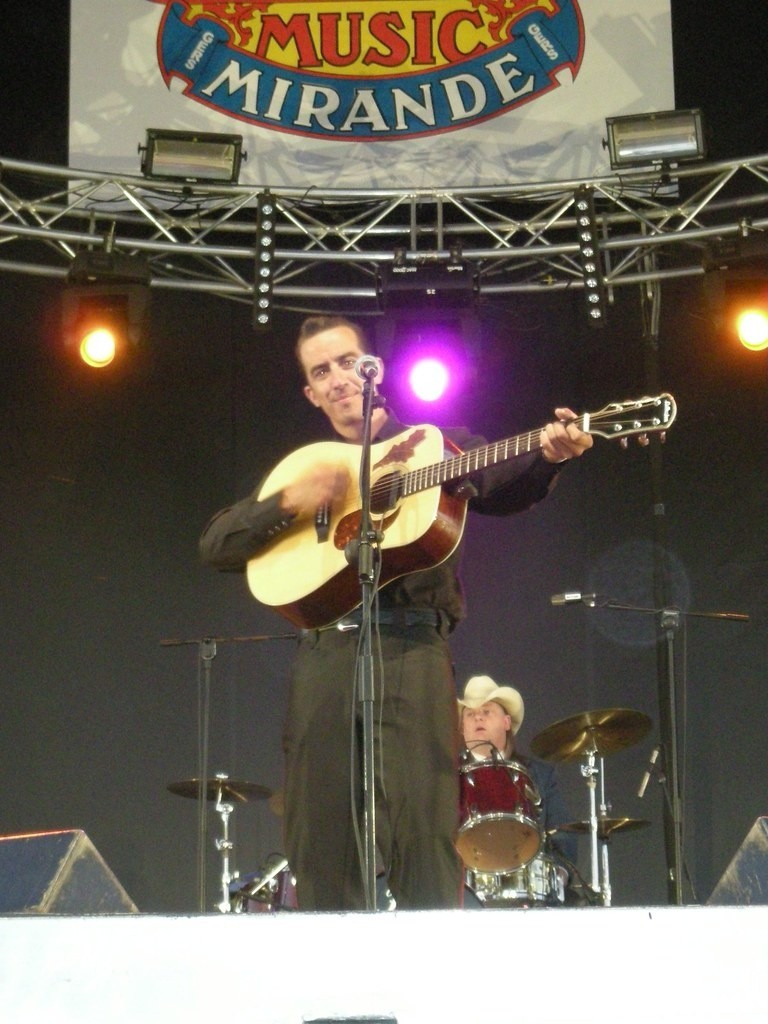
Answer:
[458,675,577,883]
[198,312,591,912]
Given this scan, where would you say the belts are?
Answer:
[298,609,440,641]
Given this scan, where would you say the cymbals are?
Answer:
[530,707,653,763]
[556,817,653,836]
[166,774,275,804]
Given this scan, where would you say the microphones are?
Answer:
[637,746,661,798]
[552,592,603,606]
[355,356,380,380]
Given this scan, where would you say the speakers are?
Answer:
[0,829,139,916]
[704,816,768,906]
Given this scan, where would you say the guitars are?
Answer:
[244,391,678,630]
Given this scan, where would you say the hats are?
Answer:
[457,675,525,737]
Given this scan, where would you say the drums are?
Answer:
[246,862,299,911]
[465,851,571,907]
[453,758,543,876]
